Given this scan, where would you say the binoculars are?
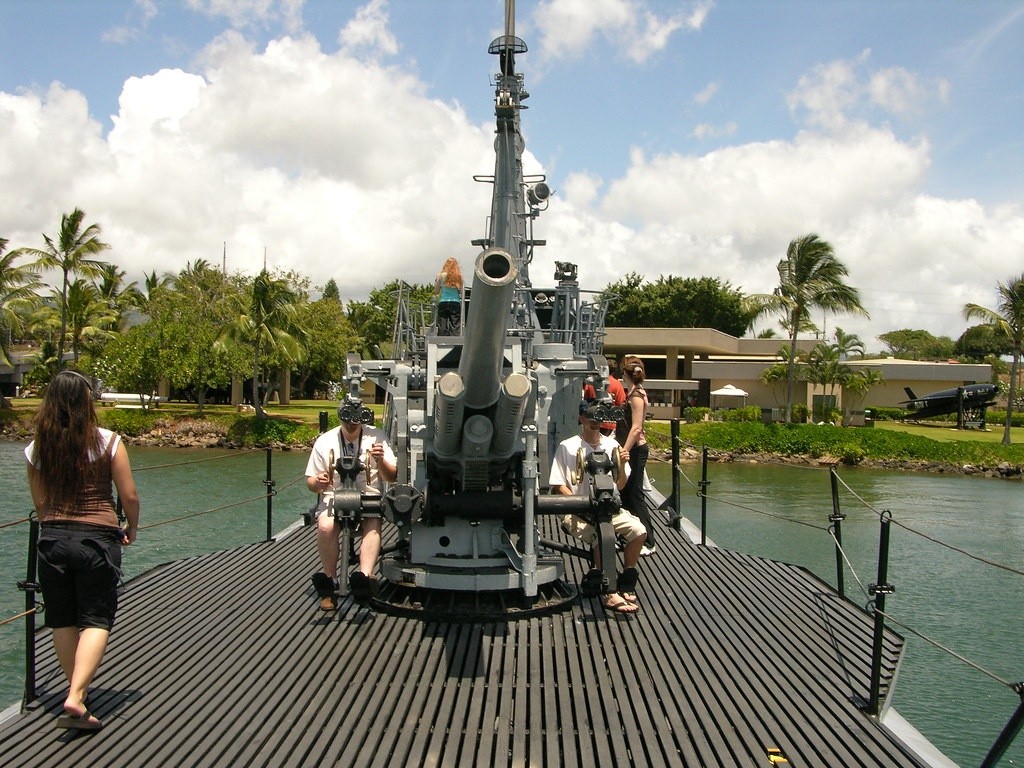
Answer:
[583,400,625,422]
[336,405,375,425]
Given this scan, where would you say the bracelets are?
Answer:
[126,522,139,532]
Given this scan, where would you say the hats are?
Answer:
[578,399,599,425]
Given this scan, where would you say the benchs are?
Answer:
[237,405,250,412]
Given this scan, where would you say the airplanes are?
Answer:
[897,383,999,411]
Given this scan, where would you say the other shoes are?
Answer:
[320,596,338,610]
[614,539,627,549]
[368,574,376,590]
[639,545,656,556]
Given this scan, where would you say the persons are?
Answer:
[305,399,397,610]
[687,394,698,407]
[435,257,464,336]
[549,397,647,614]
[584,356,656,556]
[24,369,140,729]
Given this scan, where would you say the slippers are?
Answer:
[604,597,638,613]
[65,692,89,708]
[620,589,637,603]
[56,711,102,729]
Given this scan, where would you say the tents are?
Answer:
[710,384,748,397]
